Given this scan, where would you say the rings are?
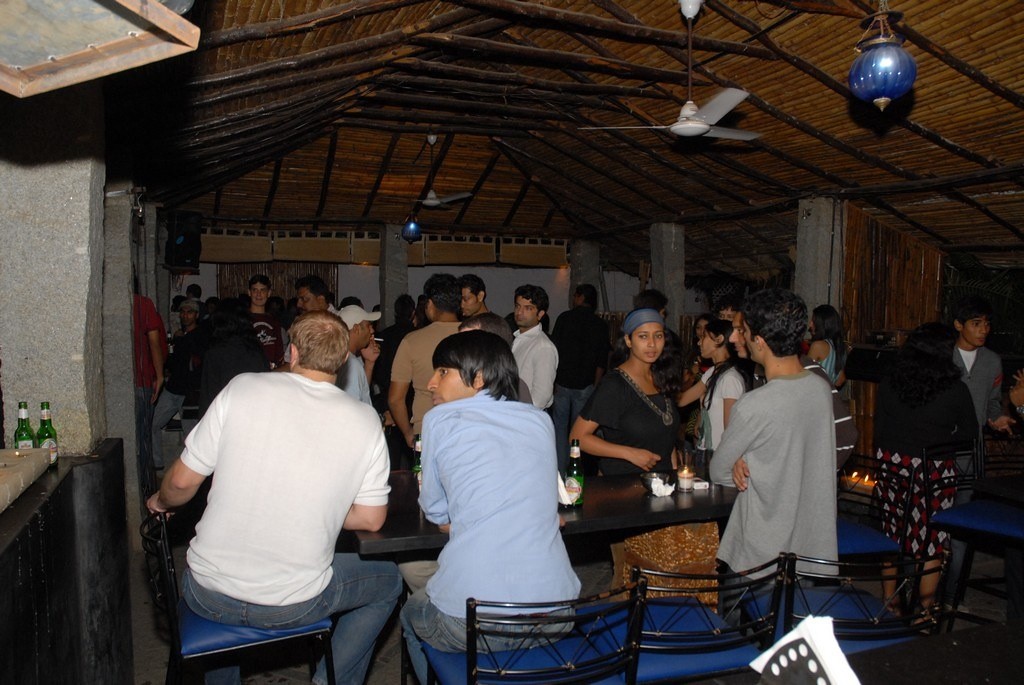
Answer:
[1010,386,1013,388]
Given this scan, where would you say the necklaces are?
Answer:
[615,367,674,425]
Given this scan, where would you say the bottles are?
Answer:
[167,330,173,354]
[411,434,422,499]
[14,402,36,449]
[565,439,584,509]
[36,402,58,470]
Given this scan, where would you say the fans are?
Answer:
[578,0,763,144]
[382,134,473,209]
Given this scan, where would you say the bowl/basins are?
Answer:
[640,472,669,492]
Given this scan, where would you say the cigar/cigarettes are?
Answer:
[370,337,384,342]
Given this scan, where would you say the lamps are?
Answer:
[401,211,422,245]
[845,0,917,112]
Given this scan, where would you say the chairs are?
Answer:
[139,504,341,685]
[575,548,791,685]
[918,434,1024,636]
[837,452,916,610]
[417,569,650,685]
[737,552,929,664]
[976,418,1024,507]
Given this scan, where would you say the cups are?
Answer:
[676,472,696,493]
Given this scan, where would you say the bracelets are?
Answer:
[1016,404,1024,414]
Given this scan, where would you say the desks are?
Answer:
[353,463,745,559]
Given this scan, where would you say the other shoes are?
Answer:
[885,598,901,617]
[943,602,970,613]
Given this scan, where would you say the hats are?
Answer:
[338,306,382,330]
[179,300,199,311]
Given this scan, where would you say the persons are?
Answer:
[1008,368,1024,421]
[148,310,404,685]
[398,328,582,685]
[872,319,978,631]
[937,299,1017,612]
[129,274,848,574]
[708,290,841,646]
